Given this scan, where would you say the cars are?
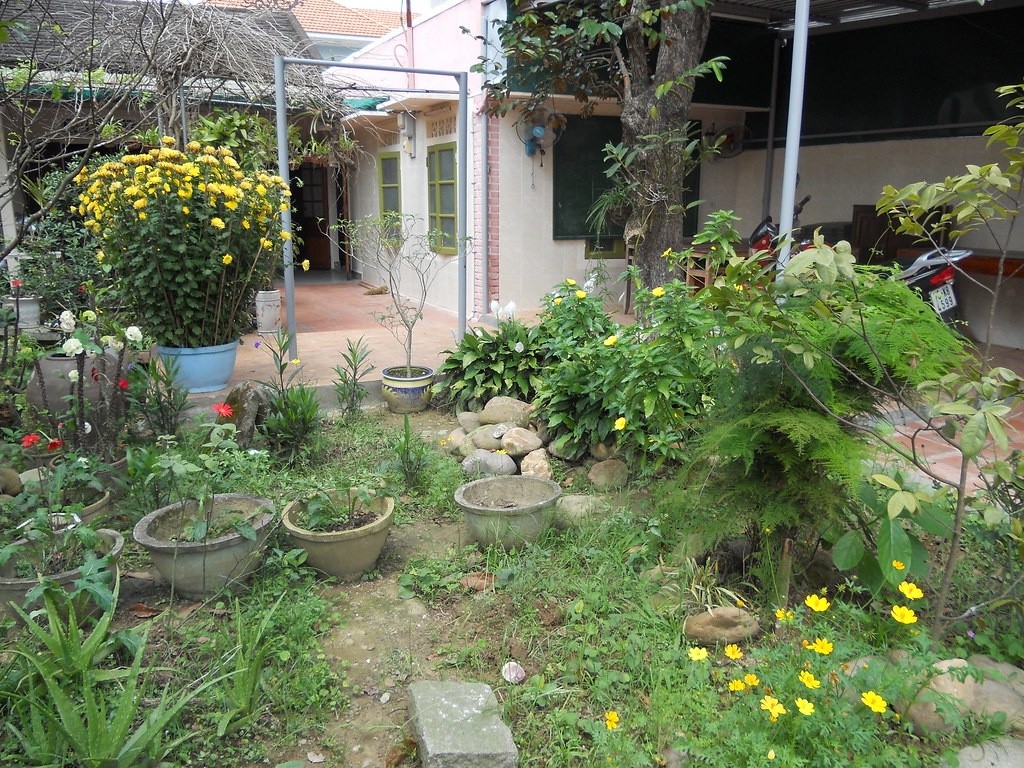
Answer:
[9,251,86,297]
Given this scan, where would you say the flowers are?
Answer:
[70,135,310,348]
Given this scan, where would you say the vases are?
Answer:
[158,340,238,391]
[454,474,563,554]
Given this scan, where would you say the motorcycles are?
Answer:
[749,195,973,334]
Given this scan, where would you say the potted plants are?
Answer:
[315,209,475,414]
[281,457,403,584]
[1,247,280,626]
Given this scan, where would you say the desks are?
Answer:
[624,236,751,312]
[895,247,1024,278]
